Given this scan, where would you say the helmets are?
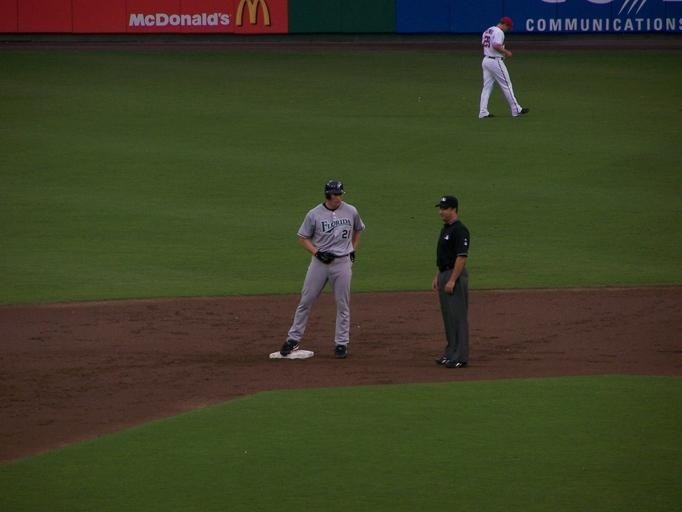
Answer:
[325,179,344,199]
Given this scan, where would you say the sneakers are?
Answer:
[280,339,299,356]
[333,345,347,358]
[482,108,529,119]
[434,354,469,368]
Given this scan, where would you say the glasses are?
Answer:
[440,206,448,209]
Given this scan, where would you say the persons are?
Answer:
[433,195,472,370]
[278,178,367,360]
[477,15,531,119]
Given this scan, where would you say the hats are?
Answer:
[435,196,458,207]
[500,16,512,26]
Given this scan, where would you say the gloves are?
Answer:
[315,251,334,264]
[350,250,355,262]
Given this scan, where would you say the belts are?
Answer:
[439,264,453,272]
[483,55,503,60]
[335,254,348,257]
